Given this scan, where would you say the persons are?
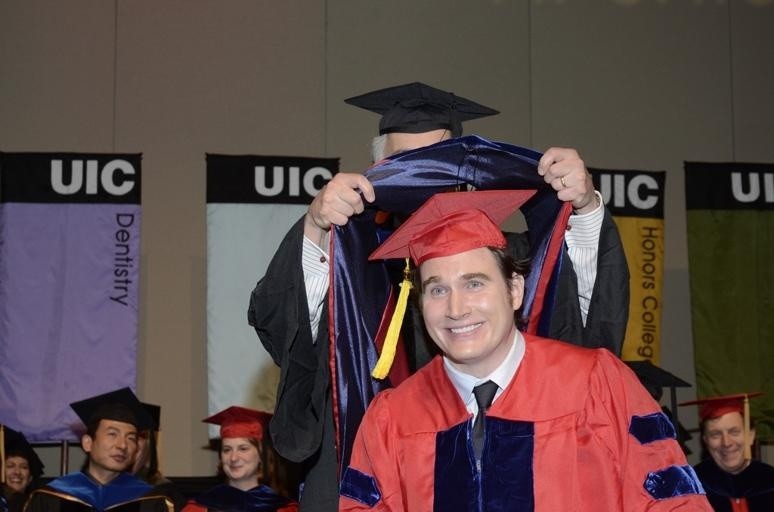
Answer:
[178,406,302,512]
[22,386,175,512]
[126,402,184,512]
[337,190,714,512]
[248,82,630,512]
[676,392,774,512]
[0,426,44,512]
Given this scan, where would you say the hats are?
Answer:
[366,186,539,271]
[67,384,165,436]
[342,81,501,139]
[622,358,693,397]
[674,387,766,421]
[200,404,273,440]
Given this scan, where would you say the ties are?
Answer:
[471,380,498,460]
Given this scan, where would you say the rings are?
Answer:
[560,175,569,189]
